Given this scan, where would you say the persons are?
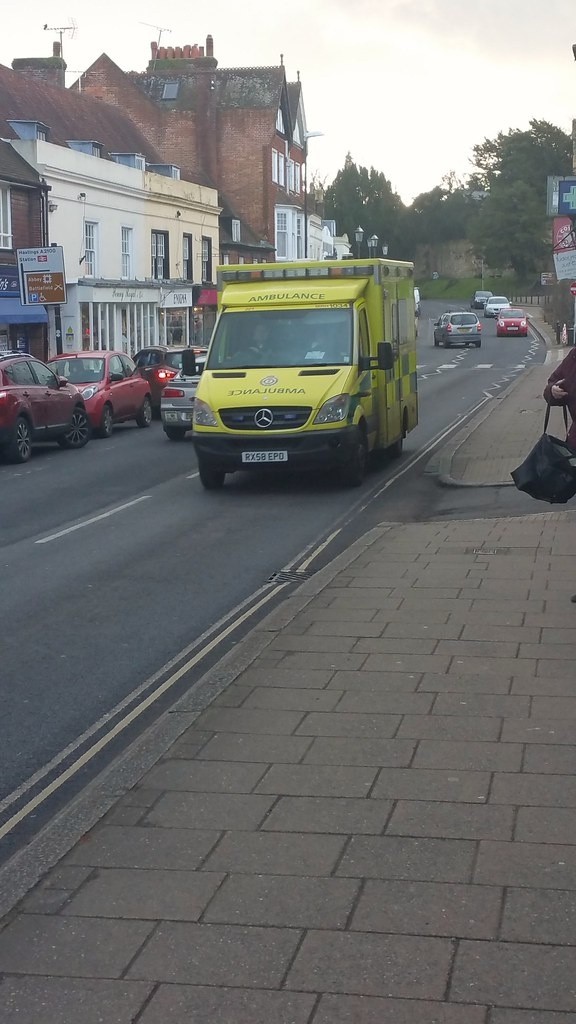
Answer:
[249,321,271,351]
[543,347,576,451]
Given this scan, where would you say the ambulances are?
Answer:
[193,256,423,486]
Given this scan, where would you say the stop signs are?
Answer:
[569,282,576,297]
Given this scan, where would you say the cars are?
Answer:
[483,296,513,318]
[44,351,151,438]
[0,350,91,463]
[131,345,208,440]
[495,308,529,338]
[470,290,495,309]
[433,312,481,353]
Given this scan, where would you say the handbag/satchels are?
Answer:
[510,403,576,504]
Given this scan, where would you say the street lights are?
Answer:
[355,225,389,260]
[302,131,324,276]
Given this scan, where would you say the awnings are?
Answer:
[0,298,49,323]
